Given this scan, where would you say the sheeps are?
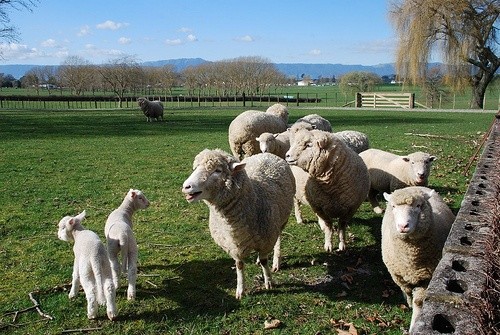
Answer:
[286,128,372,156]
[287,113,334,149]
[285,127,371,254]
[58,210,118,323]
[138,97,165,124]
[229,104,291,163]
[256,130,291,161]
[359,148,438,216]
[105,188,152,302]
[182,147,298,300]
[380,186,457,335]
